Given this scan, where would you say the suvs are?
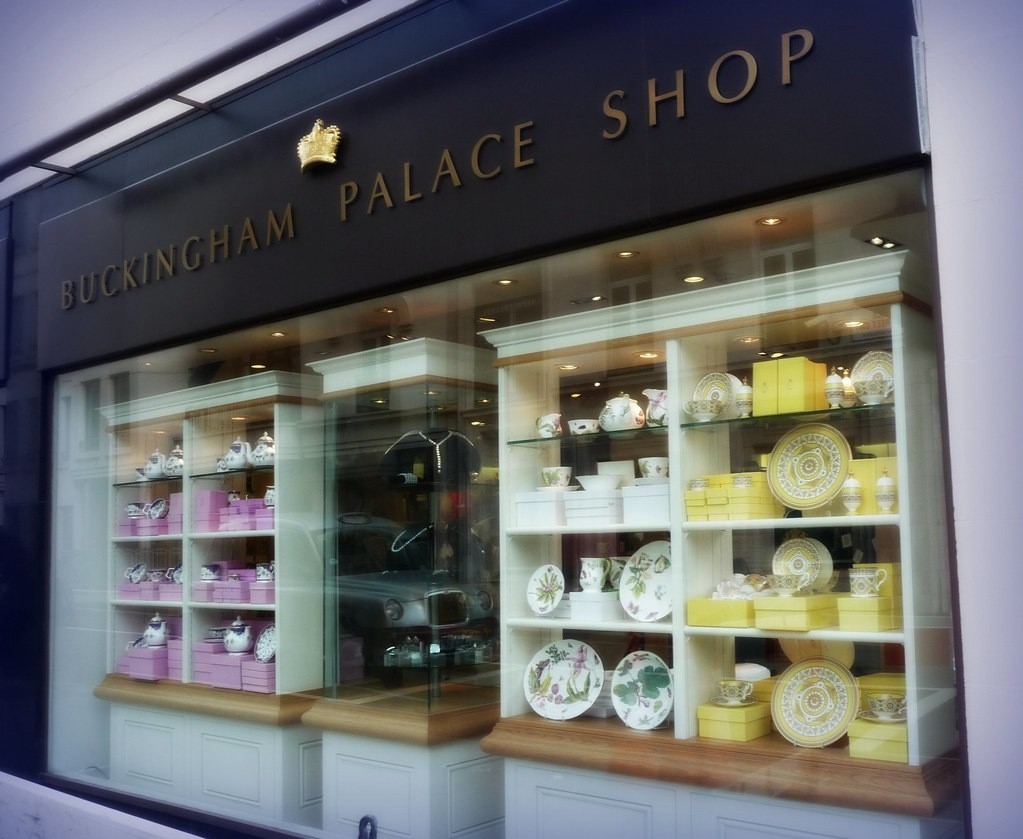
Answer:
[336,512,494,679]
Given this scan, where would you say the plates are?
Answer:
[255,626,275,662]
[766,422,852,510]
[525,541,673,729]
[632,478,670,484]
[537,485,579,492]
[804,538,831,591]
[718,373,742,420]
[857,708,908,725]
[770,656,859,748]
[772,540,820,587]
[711,695,761,708]
[692,372,733,415]
[850,350,895,400]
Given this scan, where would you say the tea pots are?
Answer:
[144,612,173,649]
[144,444,186,481]
[223,616,255,656]
[599,390,645,440]
[224,431,276,472]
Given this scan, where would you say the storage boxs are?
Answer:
[515,356,909,763]
[336,635,365,684]
[115,488,275,694]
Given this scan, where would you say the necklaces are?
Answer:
[391,525,433,552]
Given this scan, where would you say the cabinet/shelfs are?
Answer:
[93,369,339,832]
[482,248,969,839]
[298,337,505,839]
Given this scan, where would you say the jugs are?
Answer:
[641,388,669,434]
[579,557,610,591]
[609,556,630,590]
[535,414,562,442]
[264,485,275,509]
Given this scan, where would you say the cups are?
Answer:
[768,574,809,595]
[217,457,229,472]
[848,566,887,596]
[853,380,889,405]
[718,679,755,704]
[816,570,842,593]
[865,695,907,718]
[200,563,221,582]
[541,467,575,486]
[731,475,753,487]
[638,457,670,479]
[691,479,710,490]
[683,401,721,421]
[124,502,147,518]
[136,468,149,482]
[256,562,275,582]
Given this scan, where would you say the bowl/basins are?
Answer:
[575,475,623,492]
[208,627,227,639]
[146,569,168,581]
[569,418,601,444]
[148,498,170,519]
[129,563,148,585]
[172,564,183,584]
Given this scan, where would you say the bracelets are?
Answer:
[397,473,418,484]
